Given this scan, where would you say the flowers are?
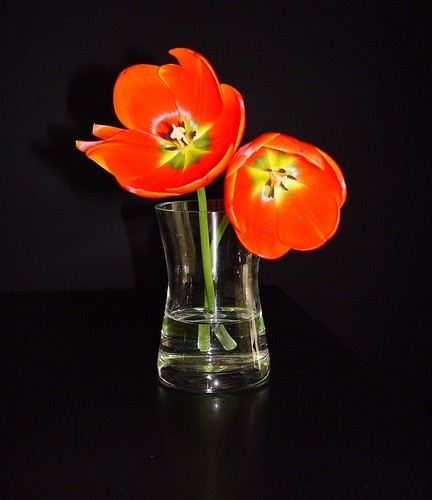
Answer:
[74,46,348,352]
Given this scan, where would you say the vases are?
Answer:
[154,200,272,394]
